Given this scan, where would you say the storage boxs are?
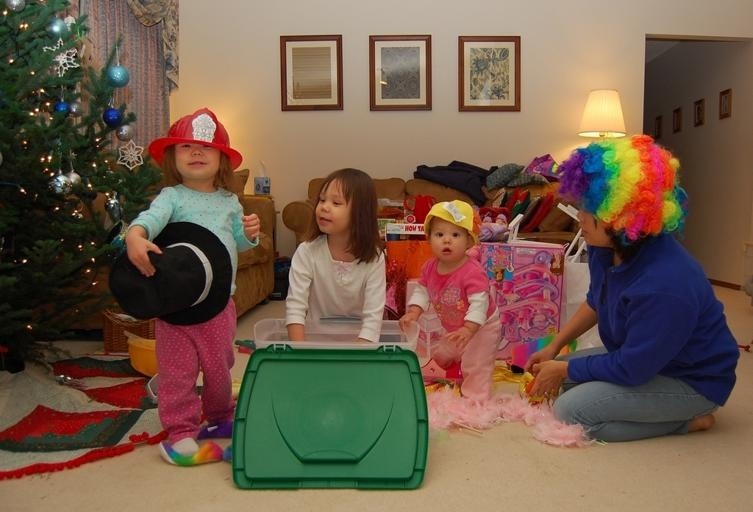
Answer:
[95,307,159,354]
[232,318,429,489]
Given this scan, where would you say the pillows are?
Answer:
[153,167,251,212]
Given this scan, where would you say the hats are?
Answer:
[110,221,232,325]
[145,107,242,172]
[424,199,480,248]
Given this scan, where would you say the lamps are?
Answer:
[577,88,627,139]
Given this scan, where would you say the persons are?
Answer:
[126,107,260,456]
[398,200,502,402]
[524,135,741,443]
[286,167,386,343]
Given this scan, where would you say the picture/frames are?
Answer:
[673,107,682,134]
[457,34,522,113]
[694,99,706,127]
[369,33,432,112]
[720,89,733,119]
[279,34,344,112]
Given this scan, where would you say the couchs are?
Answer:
[283,178,582,255]
[29,195,276,330]
